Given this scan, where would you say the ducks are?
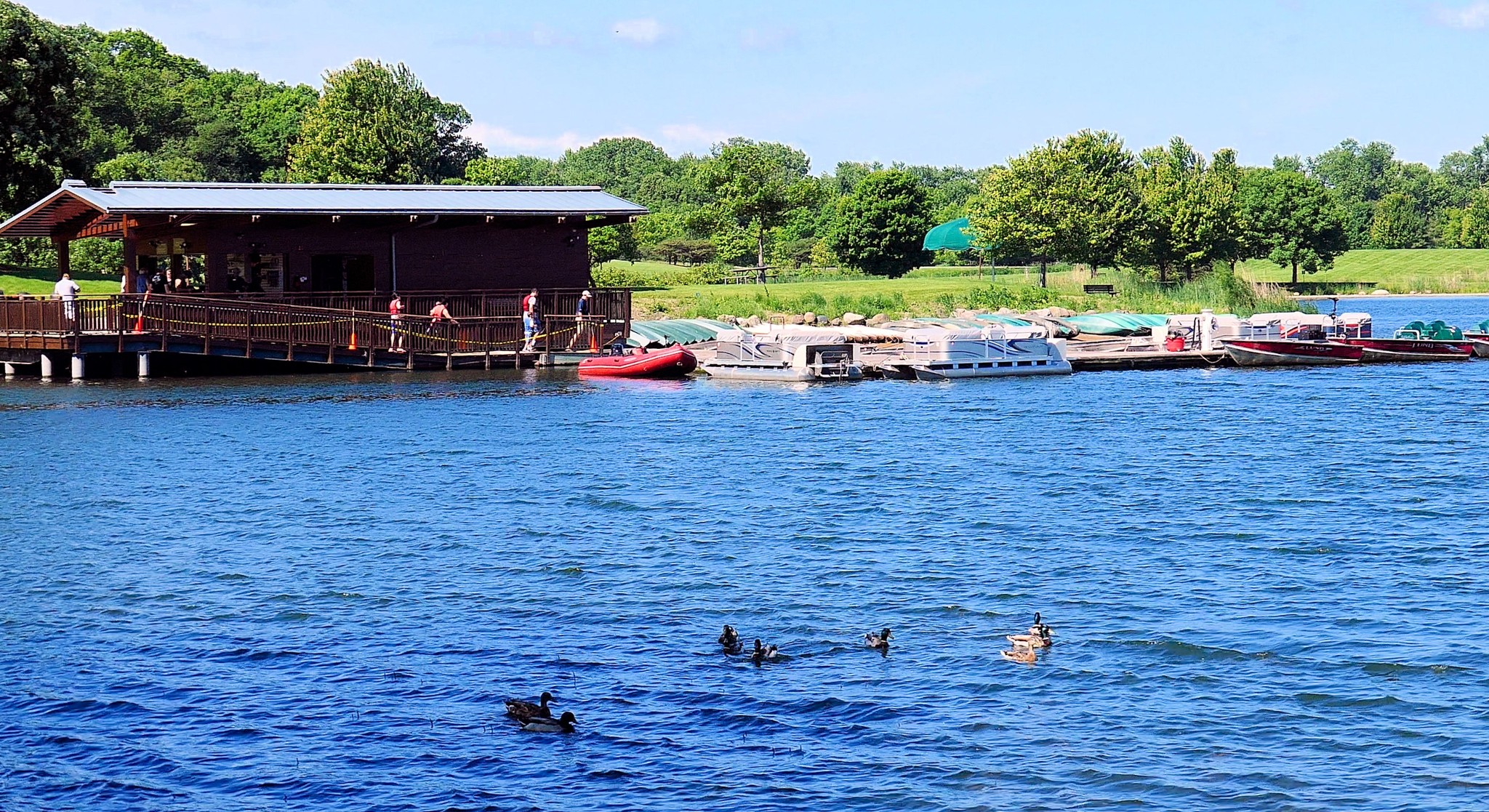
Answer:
[716,623,745,660]
[504,690,583,735]
[750,638,778,661]
[861,627,895,648]
[999,610,1058,667]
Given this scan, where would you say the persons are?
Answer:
[121,275,131,333]
[388,292,407,352]
[425,297,460,353]
[565,290,592,352]
[522,287,542,352]
[54,273,80,330]
[136,267,149,313]
[149,269,171,329]
[227,267,249,289]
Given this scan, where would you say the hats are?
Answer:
[582,290,592,298]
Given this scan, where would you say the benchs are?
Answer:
[749,274,779,283]
[721,274,753,285]
[1083,284,1120,297]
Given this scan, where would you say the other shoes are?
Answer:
[388,348,397,353]
[522,349,529,352]
[397,348,406,352]
[565,347,577,352]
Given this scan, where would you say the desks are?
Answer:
[729,267,767,285]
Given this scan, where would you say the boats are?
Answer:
[903,327,1071,381]
[1219,336,1364,368]
[684,298,1372,366]
[699,329,864,383]
[1326,319,1476,362]
[577,343,698,377]
[1462,319,1489,359]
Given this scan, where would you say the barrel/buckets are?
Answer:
[1166,337,1186,351]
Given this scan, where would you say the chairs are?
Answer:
[1397,319,1489,340]
